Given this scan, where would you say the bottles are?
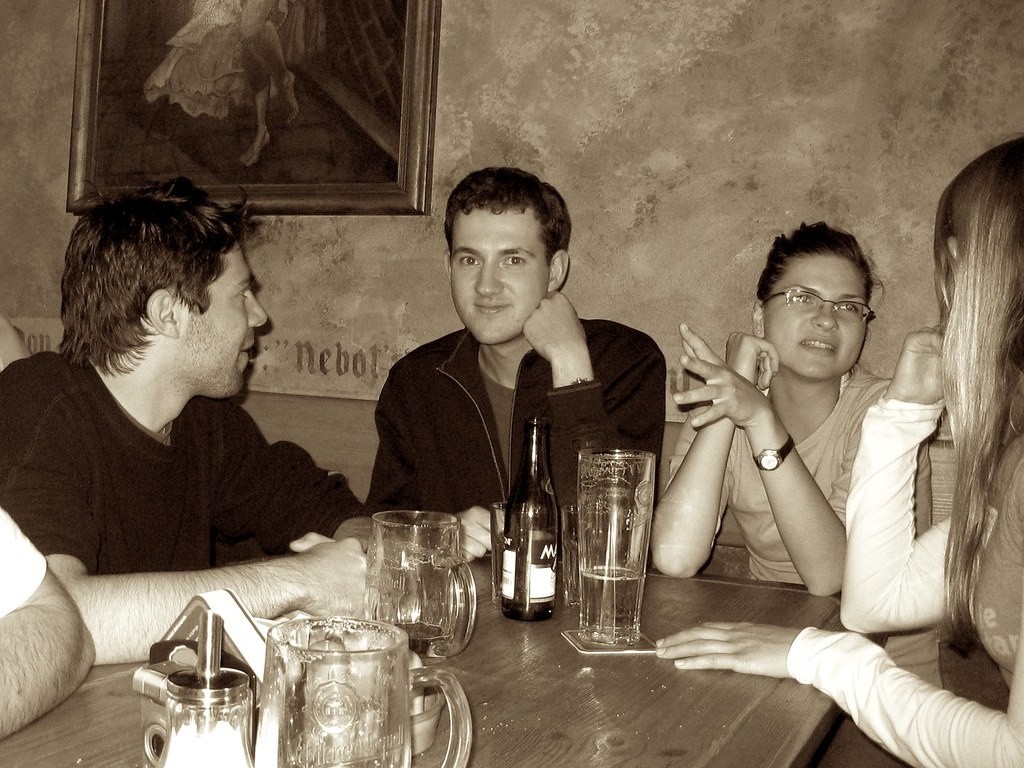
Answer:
[155,668,254,768]
[502,417,558,621]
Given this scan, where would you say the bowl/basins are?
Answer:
[410,685,446,757]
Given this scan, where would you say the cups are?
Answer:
[578,449,655,646]
[365,509,476,665]
[491,501,510,608]
[560,505,580,609]
[254,617,472,768]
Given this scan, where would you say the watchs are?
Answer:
[753,433,795,471]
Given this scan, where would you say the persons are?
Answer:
[650,221,932,598]
[0,506,95,742]
[0,176,378,667]
[654,136,1024,768]
[363,166,666,564]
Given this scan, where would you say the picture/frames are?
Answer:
[66,0,443,214]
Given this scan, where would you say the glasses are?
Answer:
[762,289,875,323]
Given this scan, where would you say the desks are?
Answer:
[1,559,890,767]
